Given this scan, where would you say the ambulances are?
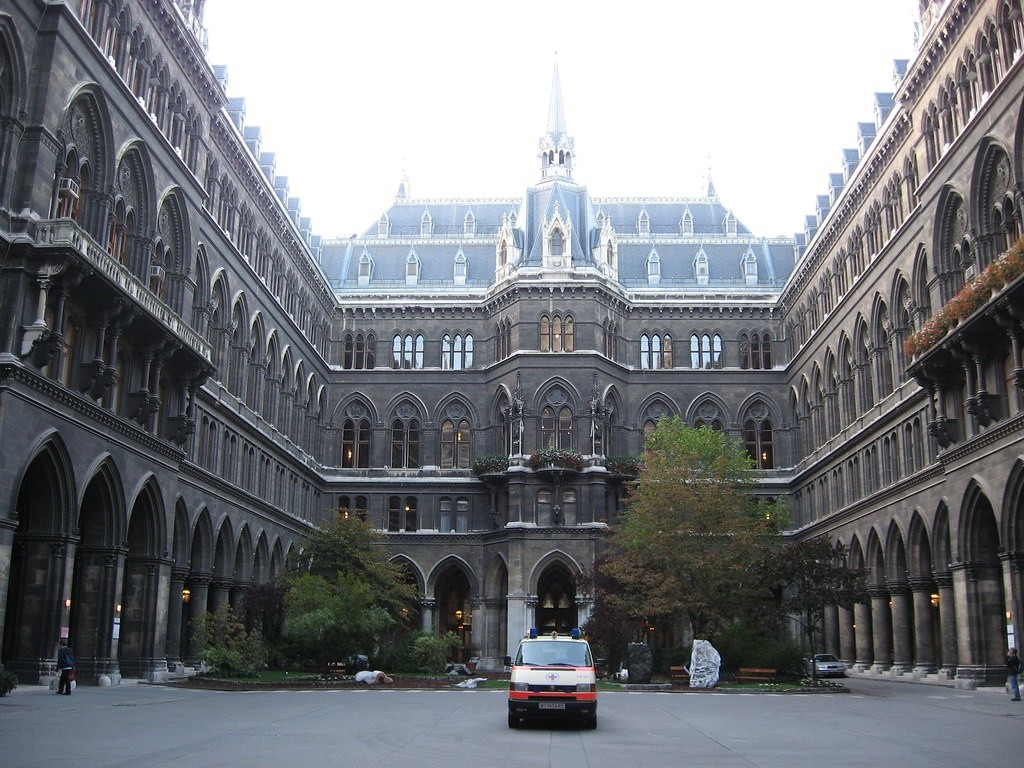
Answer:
[503,627,607,730]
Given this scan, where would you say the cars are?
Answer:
[807,652,848,679]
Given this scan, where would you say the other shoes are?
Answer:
[62,692,70,695]
[56,692,62,694]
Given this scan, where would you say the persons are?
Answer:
[56,639,75,695]
[1004,648,1021,701]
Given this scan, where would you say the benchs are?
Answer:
[733,666,778,684]
[669,666,689,684]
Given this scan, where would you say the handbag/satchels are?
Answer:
[69,669,76,681]
